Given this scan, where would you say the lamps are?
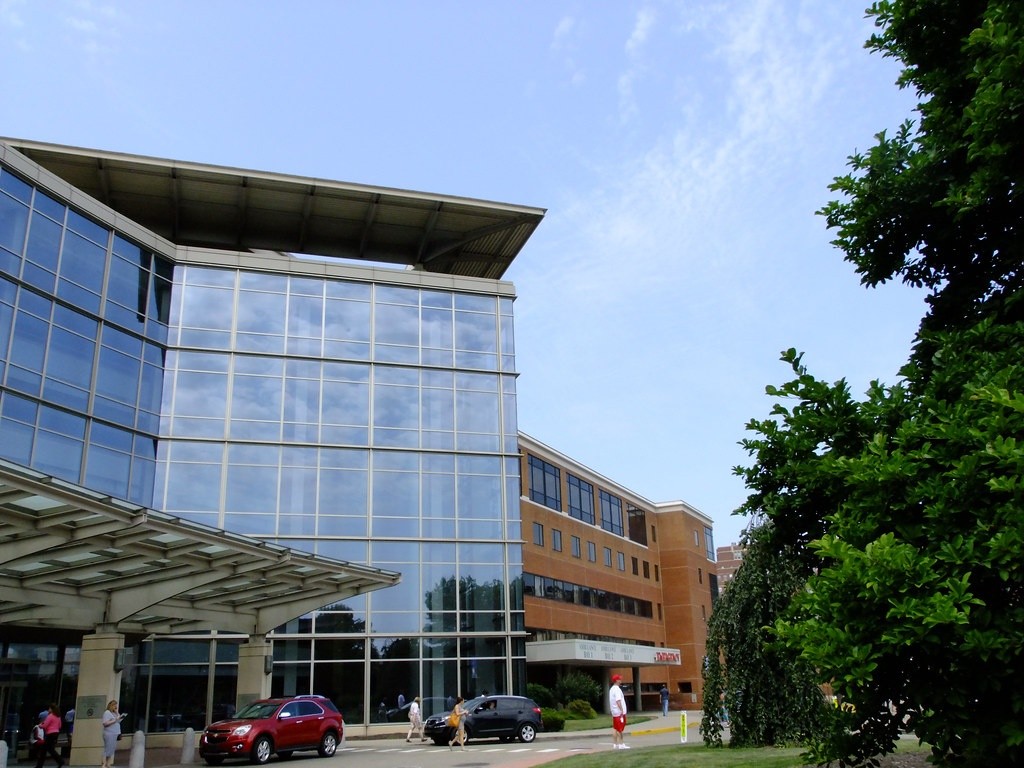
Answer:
[264,655,273,675]
[114,649,127,673]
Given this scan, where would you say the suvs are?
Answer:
[385,697,460,724]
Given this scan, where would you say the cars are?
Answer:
[423,695,545,745]
[199,695,346,765]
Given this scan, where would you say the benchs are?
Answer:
[27,741,71,758]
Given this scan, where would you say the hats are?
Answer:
[611,674,624,683]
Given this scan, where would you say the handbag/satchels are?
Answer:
[448,712,461,728]
[117,734,121,740]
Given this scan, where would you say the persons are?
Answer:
[28,703,75,768]
[717,688,731,728]
[406,697,427,743]
[485,702,495,711]
[102,700,121,768]
[660,684,669,716]
[609,675,631,749]
[398,692,406,708]
[448,697,468,751]
[476,688,490,709]
[379,701,388,723]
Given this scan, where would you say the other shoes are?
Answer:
[58,762,65,768]
[34,767,42,768]
[406,739,411,742]
[449,741,453,751]
[461,749,468,751]
[421,739,427,742]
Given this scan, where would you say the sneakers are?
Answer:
[613,743,631,750]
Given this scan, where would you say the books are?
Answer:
[118,713,128,722]
